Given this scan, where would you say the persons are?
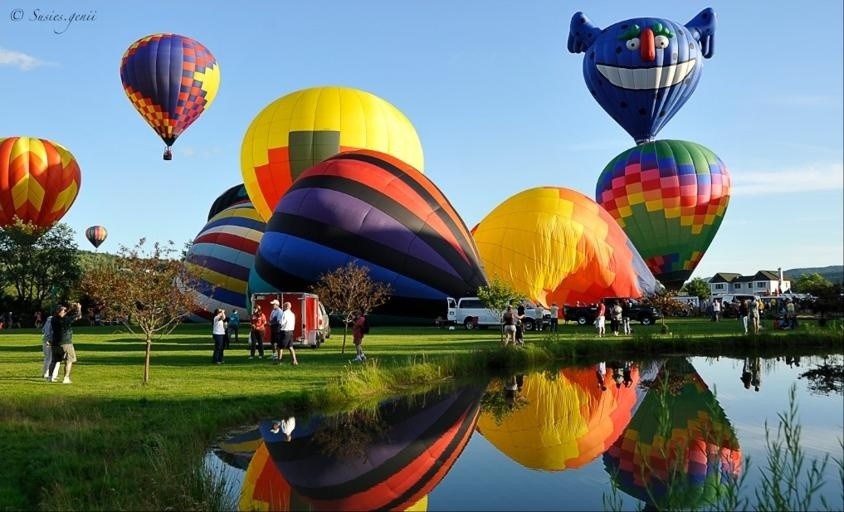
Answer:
[212,299,299,365]
[350,307,367,361]
[740,355,800,391]
[0,303,128,385]
[502,298,631,346]
[684,296,817,335]
[269,416,296,443]
[436,316,443,328]
[498,357,634,410]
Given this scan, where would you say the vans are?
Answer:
[436,298,553,332]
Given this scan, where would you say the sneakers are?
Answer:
[267,353,279,360]
[352,354,367,362]
[62,377,73,384]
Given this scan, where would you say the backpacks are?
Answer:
[360,315,370,334]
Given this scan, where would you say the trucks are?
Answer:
[244,292,330,351]
[714,289,819,317]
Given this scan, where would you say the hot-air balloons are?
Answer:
[562,3,719,151]
[0,135,83,254]
[85,225,108,252]
[116,31,223,163]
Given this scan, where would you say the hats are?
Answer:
[269,299,280,306]
